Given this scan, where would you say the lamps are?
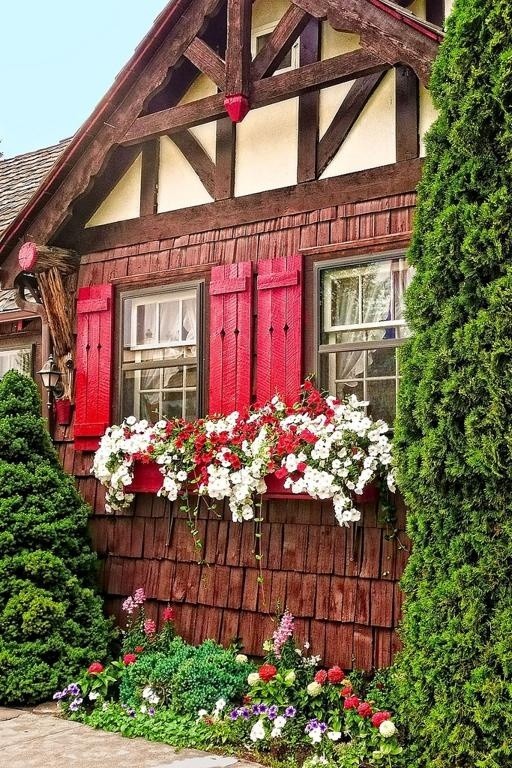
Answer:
[35,353,64,412]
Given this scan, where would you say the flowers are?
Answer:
[90,382,396,541]
